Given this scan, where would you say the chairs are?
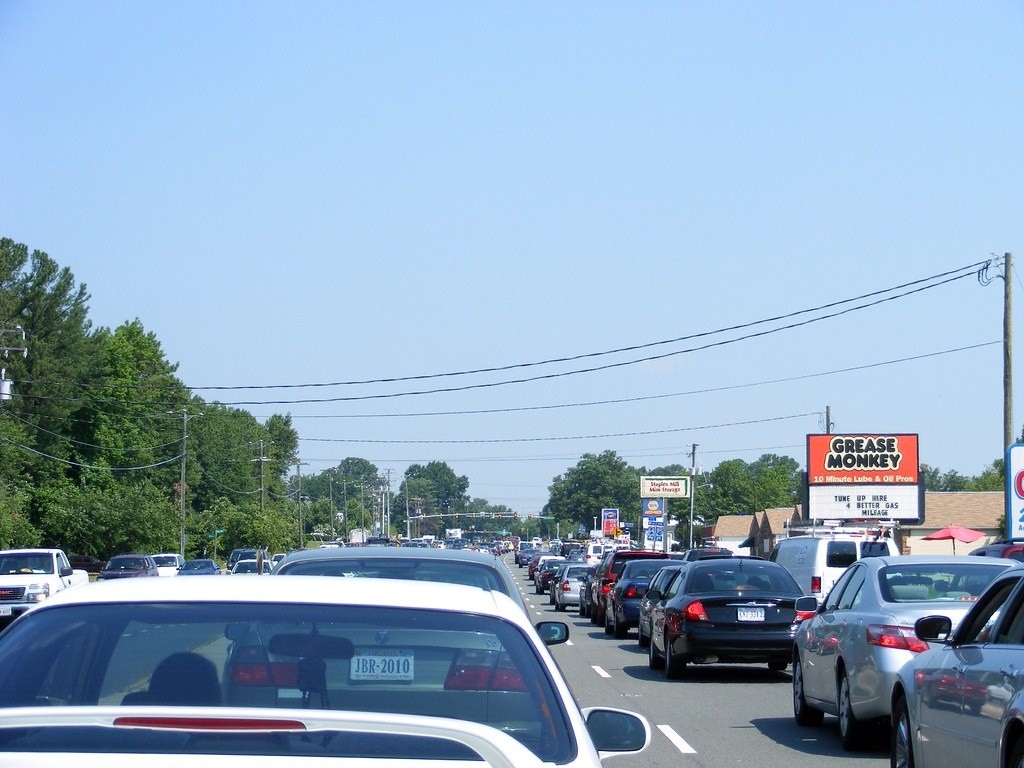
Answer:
[889,585,929,598]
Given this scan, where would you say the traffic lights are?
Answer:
[529,514,531,519]
[498,514,502,520]
[514,512,518,521]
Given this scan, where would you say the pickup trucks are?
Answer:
[0,550,89,615]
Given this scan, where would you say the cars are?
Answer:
[514,539,1024,768]
[913,664,989,717]
[0,539,651,768]
[68,555,107,574]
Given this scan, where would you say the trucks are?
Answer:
[447,528,462,540]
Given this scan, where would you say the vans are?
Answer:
[768,536,902,612]
